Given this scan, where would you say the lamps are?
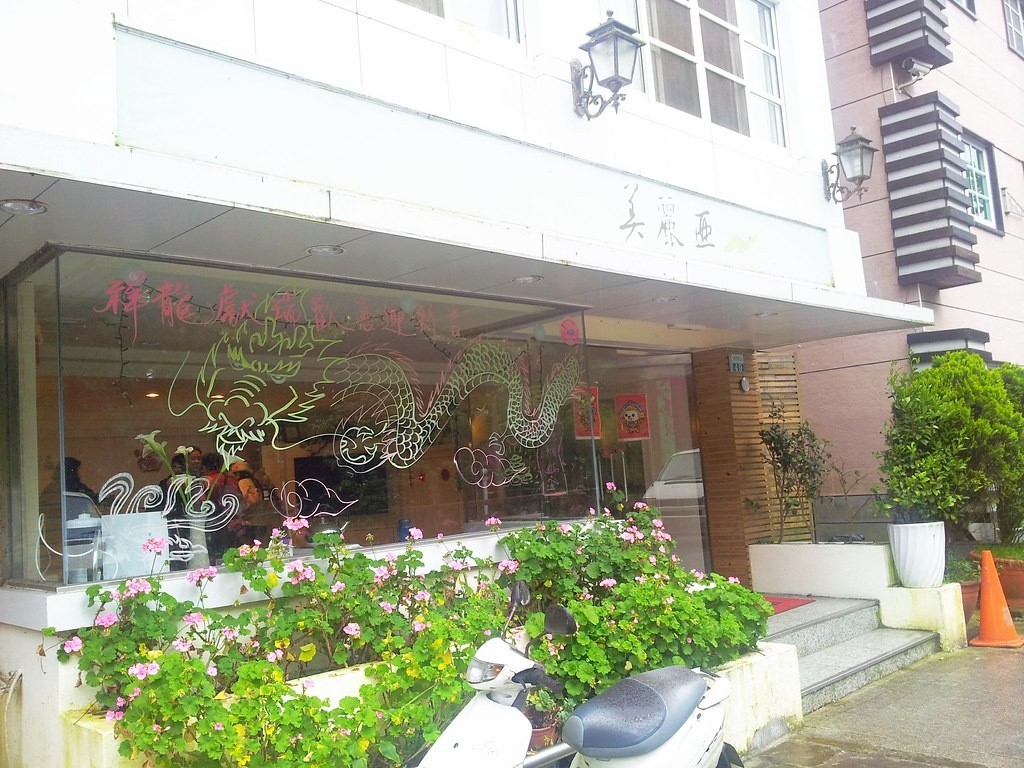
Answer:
[820,125,880,205]
[569,9,647,121]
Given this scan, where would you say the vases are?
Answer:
[521,689,561,751]
[182,514,214,570]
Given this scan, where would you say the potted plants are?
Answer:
[869,349,993,588]
[968,362,1024,619]
[917,349,1023,625]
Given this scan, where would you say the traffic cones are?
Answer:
[966,550,1024,648]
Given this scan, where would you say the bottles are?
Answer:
[398,520,412,543]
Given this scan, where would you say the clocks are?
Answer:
[741,376,750,391]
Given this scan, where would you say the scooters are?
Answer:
[396,579,744,768]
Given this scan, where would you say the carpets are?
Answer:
[753,596,816,617]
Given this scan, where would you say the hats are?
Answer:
[232,461,253,473]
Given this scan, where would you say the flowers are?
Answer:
[134,429,246,513]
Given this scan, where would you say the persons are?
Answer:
[40,457,121,550]
[158,448,277,554]
